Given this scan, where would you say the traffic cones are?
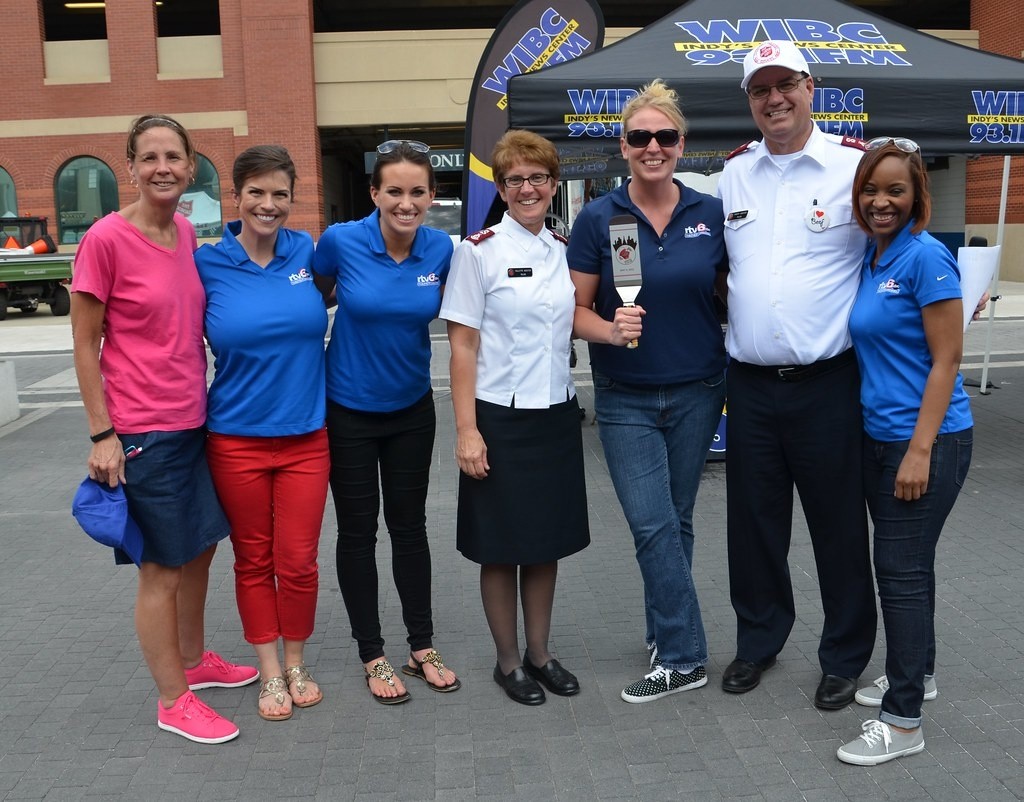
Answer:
[23,235,57,254]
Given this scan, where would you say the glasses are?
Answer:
[376,139,431,160]
[624,129,680,147]
[747,76,806,100]
[863,137,921,161]
[502,174,552,188]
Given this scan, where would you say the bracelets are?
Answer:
[90,424,115,442]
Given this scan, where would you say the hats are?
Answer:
[72,476,144,568]
[740,40,811,94]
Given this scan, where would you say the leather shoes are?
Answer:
[523,648,581,695]
[814,674,857,707]
[493,661,545,705]
[723,655,776,693]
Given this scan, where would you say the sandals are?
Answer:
[401,650,461,692]
[284,665,323,707]
[363,660,411,704]
[258,674,293,721]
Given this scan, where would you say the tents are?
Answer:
[506,0,1024,392]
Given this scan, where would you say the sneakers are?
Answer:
[620,666,707,704]
[837,720,924,765]
[856,674,937,706]
[646,641,662,668]
[157,689,239,743]
[184,651,260,690]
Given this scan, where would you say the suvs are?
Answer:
[421,197,463,252]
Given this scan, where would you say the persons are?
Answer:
[71,115,259,744]
[567,84,726,702]
[718,41,989,709]
[195,145,338,721]
[438,131,592,705]
[312,141,460,704]
[837,143,975,767]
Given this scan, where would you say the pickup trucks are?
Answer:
[0,217,73,321]
